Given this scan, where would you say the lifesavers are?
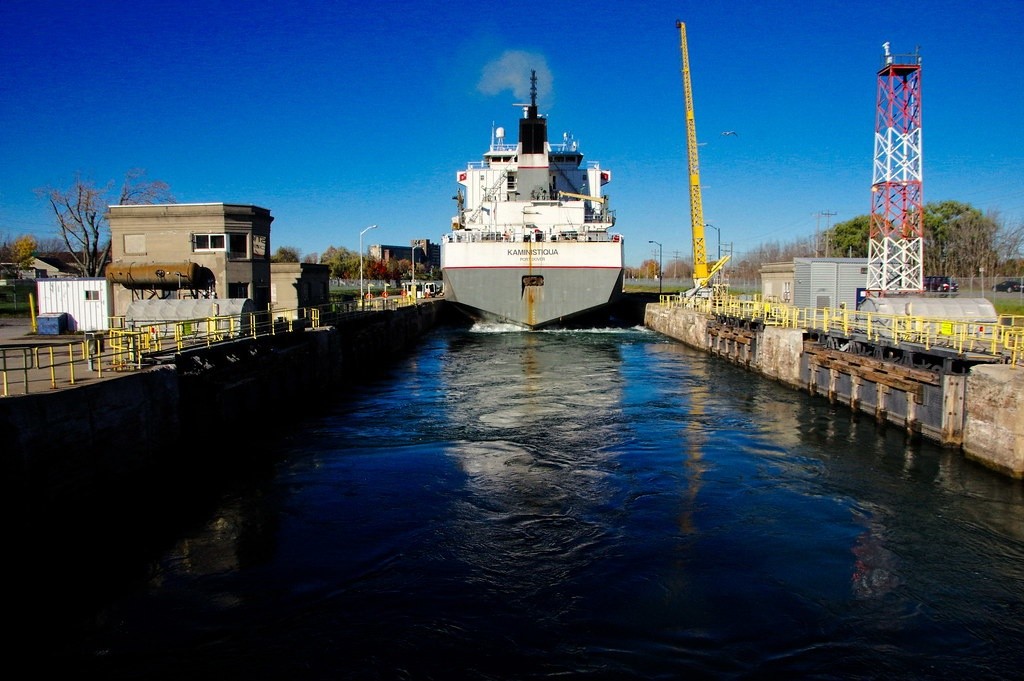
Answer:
[366,294,373,300]
[381,291,389,298]
[401,290,407,296]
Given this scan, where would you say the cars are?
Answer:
[424,283,437,297]
[992,281,1023,293]
[925,275,958,293]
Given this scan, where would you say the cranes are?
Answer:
[678,22,714,295]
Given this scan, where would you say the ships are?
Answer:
[440,68,625,330]
